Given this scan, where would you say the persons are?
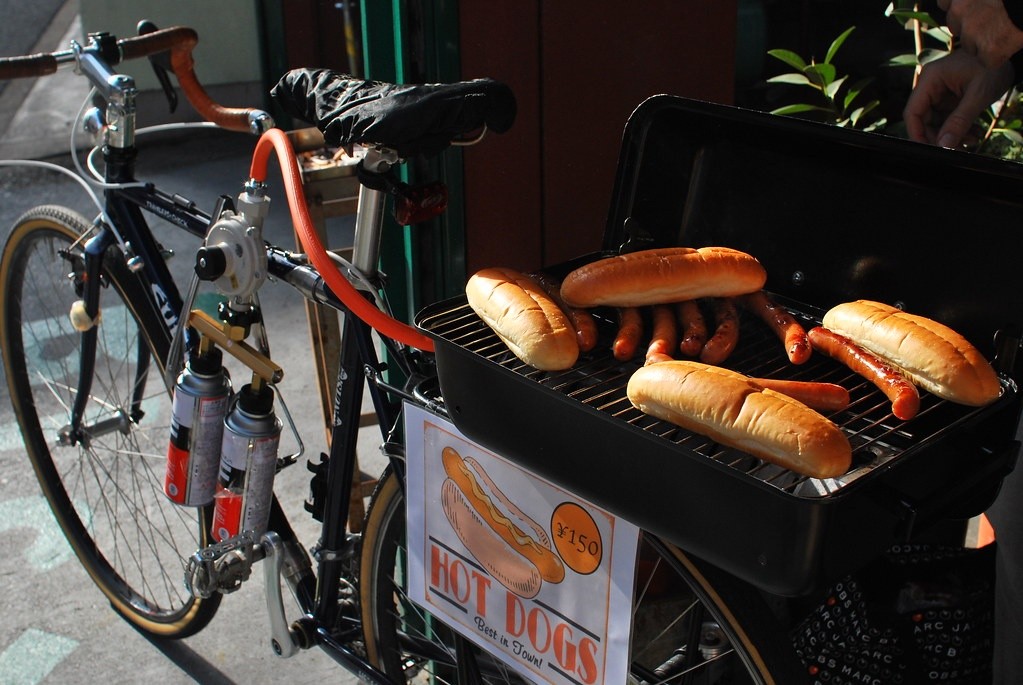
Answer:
[904,0,1023,148]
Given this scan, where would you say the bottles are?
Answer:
[166,347,284,550]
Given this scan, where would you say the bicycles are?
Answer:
[1,21,796,685]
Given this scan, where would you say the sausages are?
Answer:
[535,273,850,411]
[806,327,920,419]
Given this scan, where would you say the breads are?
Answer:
[558,246,766,308]
[465,266,579,371]
[627,360,851,480]
[822,299,1001,405]
